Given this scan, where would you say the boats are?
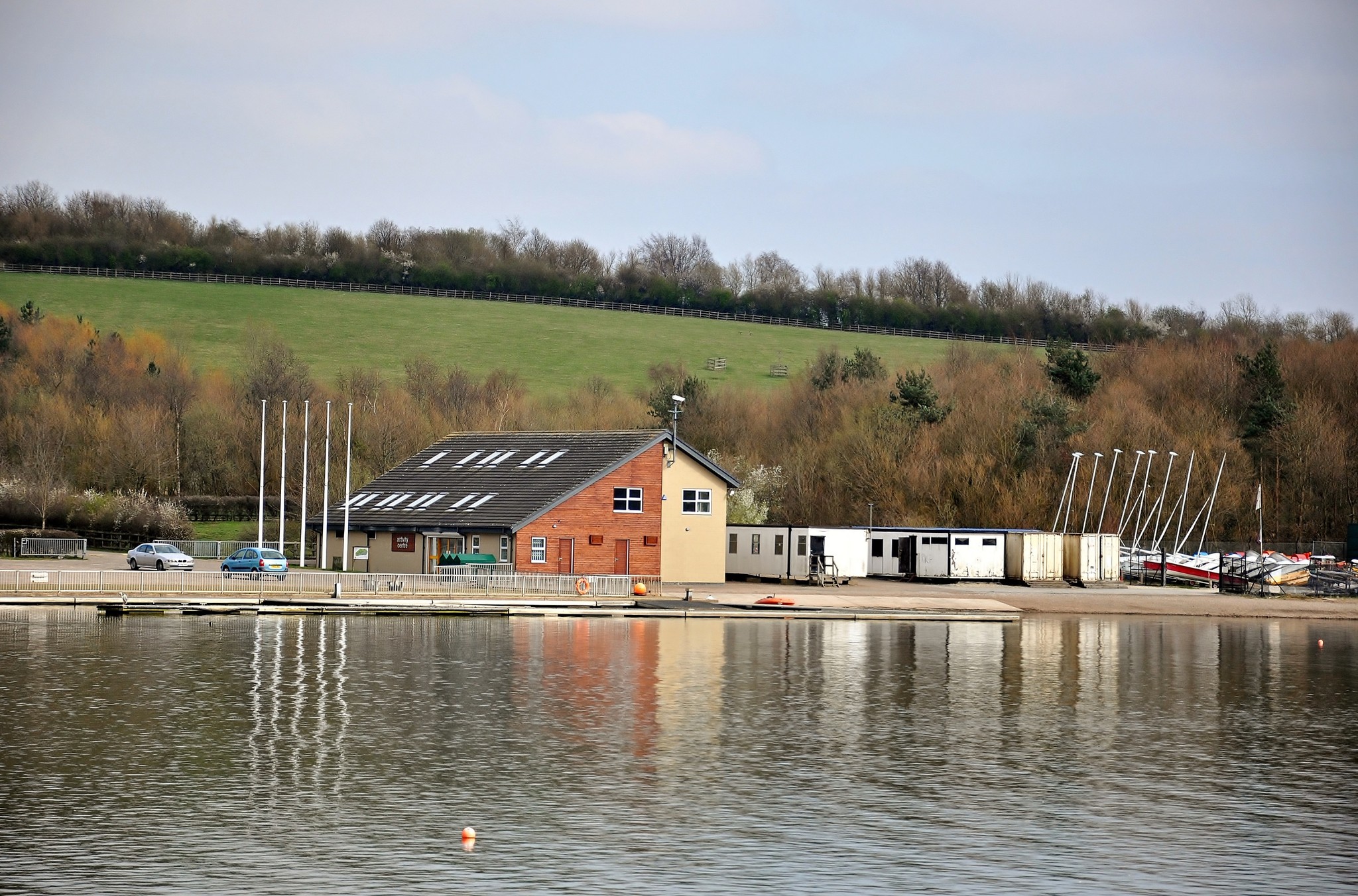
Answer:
[754,593,795,606]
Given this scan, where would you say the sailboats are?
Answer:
[1116,449,1358,600]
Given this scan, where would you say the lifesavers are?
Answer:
[576,578,589,595]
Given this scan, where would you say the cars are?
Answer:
[220,548,289,581]
[126,543,194,572]
